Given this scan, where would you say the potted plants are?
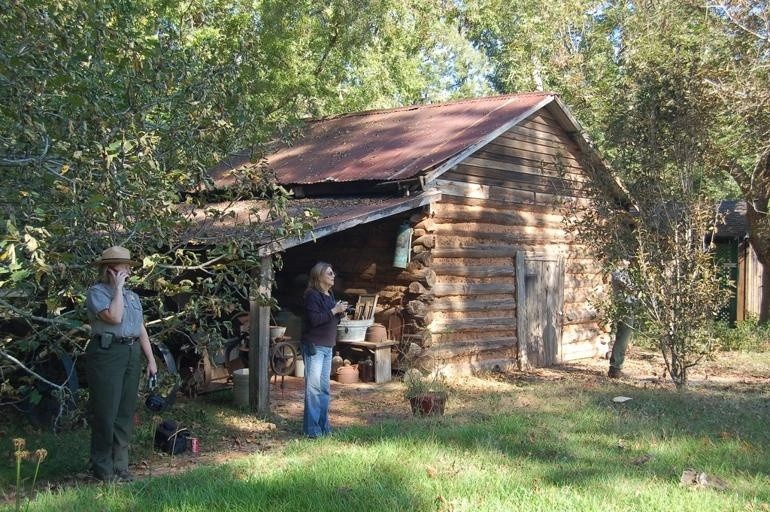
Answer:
[403,372,451,416]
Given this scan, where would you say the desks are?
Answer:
[337,338,401,384]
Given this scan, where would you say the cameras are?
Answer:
[340,303,355,315]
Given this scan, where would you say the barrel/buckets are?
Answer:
[231,367,251,409]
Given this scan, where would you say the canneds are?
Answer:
[190,438,199,453]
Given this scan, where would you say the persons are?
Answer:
[85,245,158,488]
[300,262,349,438]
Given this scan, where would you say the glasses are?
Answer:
[326,272,337,276]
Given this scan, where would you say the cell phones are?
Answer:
[107,267,128,282]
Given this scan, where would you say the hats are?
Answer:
[96,246,142,268]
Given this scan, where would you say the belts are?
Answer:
[91,333,139,346]
